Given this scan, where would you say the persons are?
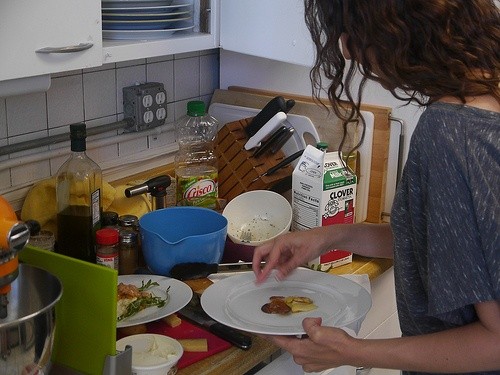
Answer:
[252,1,500,375]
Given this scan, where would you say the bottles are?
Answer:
[55,123,103,263]
[173,101,218,210]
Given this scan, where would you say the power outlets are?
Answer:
[123,81,168,131]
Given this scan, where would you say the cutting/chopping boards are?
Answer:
[207,86,404,224]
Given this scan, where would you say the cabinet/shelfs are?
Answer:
[0,1,349,80]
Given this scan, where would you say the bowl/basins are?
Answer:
[139,206,228,279]
[221,190,292,246]
[224,237,255,263]
[116,334,183,375]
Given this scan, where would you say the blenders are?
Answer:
[0,195,64,375]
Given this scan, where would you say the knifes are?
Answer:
[243,96,305,183]
[177,307,252,349]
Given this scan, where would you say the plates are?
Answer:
[117,275,194,328]
[101,0,194,39]
[200,268,372,336]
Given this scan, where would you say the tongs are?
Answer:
[169,261,268,280]
